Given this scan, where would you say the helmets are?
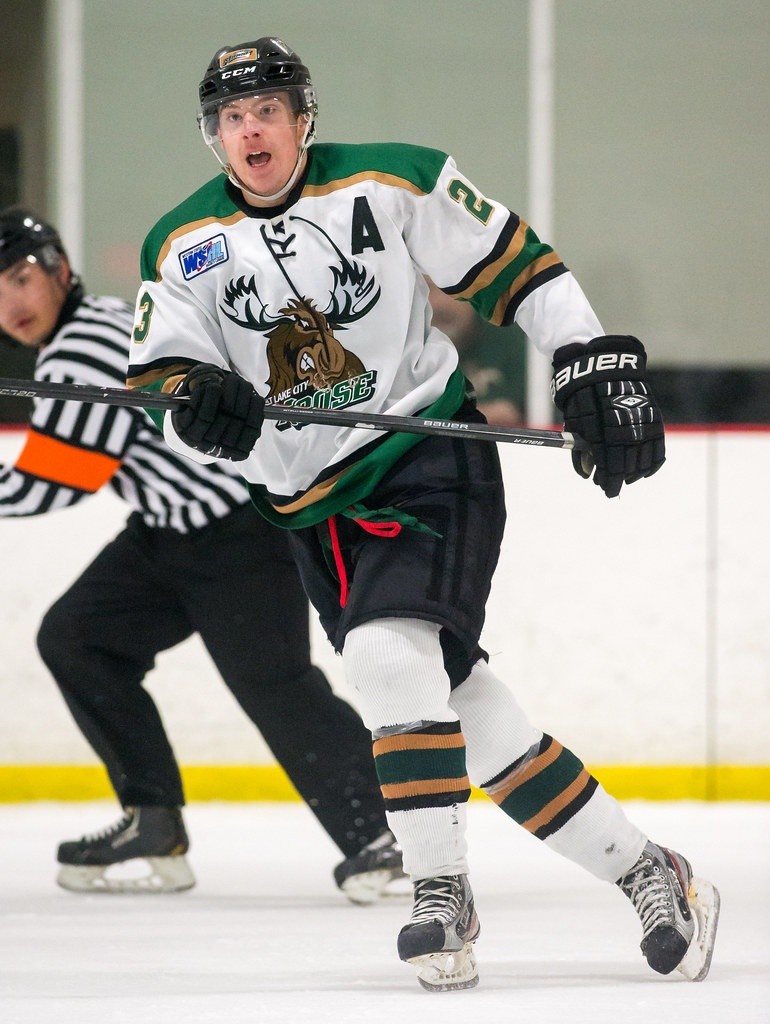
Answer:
[0,210,57,272]
[196,36,319,136]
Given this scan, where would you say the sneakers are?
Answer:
[616,840,721,982]
[334,830,415,906]
[54,806,198,895]
[397,873,481,992]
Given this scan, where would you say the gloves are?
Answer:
[170,362,266,462]
[548,335,666,498]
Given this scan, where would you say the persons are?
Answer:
[0,208,418,905]
[125,38,720,992]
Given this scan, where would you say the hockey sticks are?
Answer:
[2,374,581,458]
